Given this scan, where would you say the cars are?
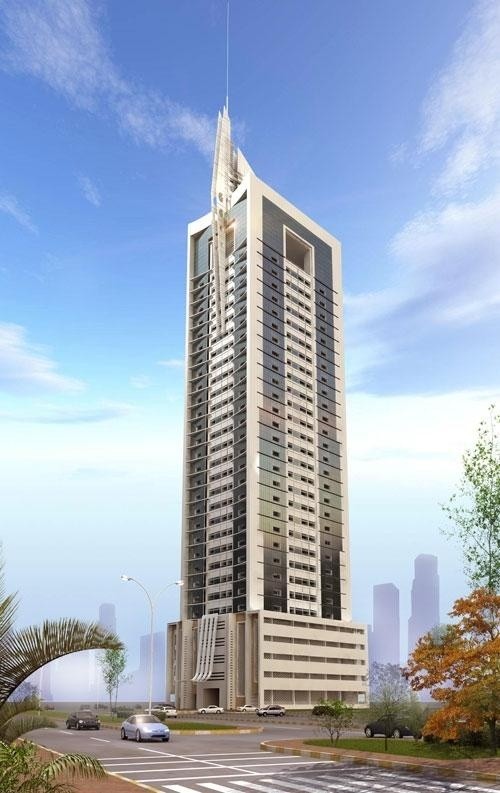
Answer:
[237,704,260,712]
[198,705,224,714]
[66,710,101,729]
[256,705,285,717]
[120,714,170,741]
[364,714,422,740]
[145,704,177,718]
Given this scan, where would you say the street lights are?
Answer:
[121,575,184,717]
